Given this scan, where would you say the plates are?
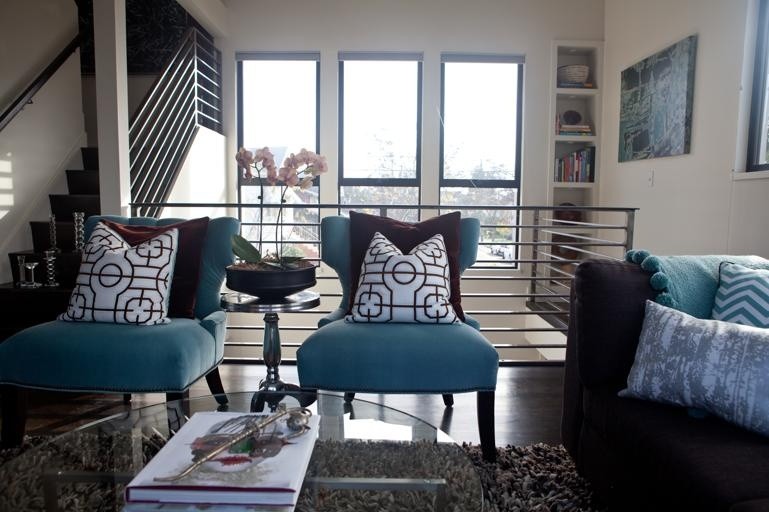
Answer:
[542,40,605,286]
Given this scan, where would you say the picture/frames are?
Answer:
[220,292,320,391]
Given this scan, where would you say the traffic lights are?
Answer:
[617,260,769,436]
[57,216,210,326]
[349,210,466,327]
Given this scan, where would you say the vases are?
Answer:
[230,146,327,271]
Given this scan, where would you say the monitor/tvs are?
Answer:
[554,112,595,183]
[559,83,593,89]
[124,409,322,505]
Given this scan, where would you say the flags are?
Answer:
[225,264,318,300]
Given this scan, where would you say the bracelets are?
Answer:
[557,63,590,84]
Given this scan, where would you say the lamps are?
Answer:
[1,215,242,439]
[295,216,500,463]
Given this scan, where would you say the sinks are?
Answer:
[560,257,768,511]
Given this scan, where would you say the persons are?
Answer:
[140,413,292,489]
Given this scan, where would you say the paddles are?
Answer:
[1,430,592,511]
